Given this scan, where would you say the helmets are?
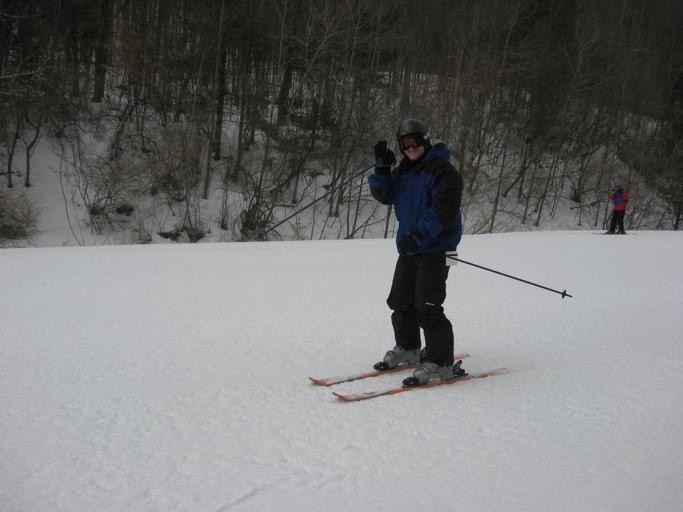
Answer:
[396,118,431,156]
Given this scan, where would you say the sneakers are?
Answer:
[384,346,421,369]
[412,361,455,384]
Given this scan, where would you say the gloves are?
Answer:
[399,233,418,251]
[374,139,390,163]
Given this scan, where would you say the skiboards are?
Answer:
[593,232,638,235]
[308,353,507,401]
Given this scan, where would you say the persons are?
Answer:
[606,183,629,234]
[367,118,464,389]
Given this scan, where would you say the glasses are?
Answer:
[398,129,430,151]
